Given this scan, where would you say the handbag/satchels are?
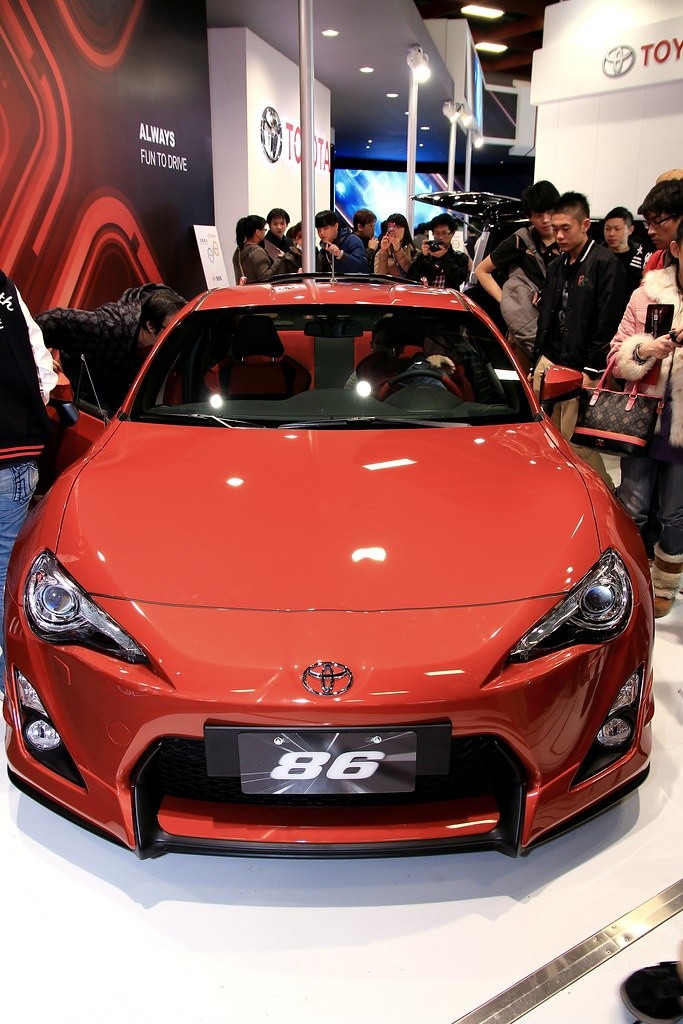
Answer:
[570,356,664,457]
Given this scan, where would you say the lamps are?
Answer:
[407,45,431,82]
[443,101,484,147]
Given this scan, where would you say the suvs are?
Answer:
[412,188,654,300]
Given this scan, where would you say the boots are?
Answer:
[651,542,683,617]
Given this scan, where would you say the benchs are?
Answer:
[274,329,419,377]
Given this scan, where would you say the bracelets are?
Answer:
[636,344,646,361]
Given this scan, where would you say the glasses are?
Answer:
[428,336,456,352]
[643,215,673,229]
[432,232,452,238]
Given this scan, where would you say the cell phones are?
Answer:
[388,222,396,238]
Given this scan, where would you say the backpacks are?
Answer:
[500,228,547,353]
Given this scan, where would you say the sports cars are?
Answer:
[3,270,658,858]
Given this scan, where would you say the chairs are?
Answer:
[227,314,312,402]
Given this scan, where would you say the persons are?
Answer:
[525,191,627,494]
[29,283,188,512]
[606,221,683,618]
[0,268,59,701]
[636,178,683,568]
[232,179,654,402]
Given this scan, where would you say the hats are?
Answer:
[425,319,464,348]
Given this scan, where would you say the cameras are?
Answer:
[425,242,444,252]
